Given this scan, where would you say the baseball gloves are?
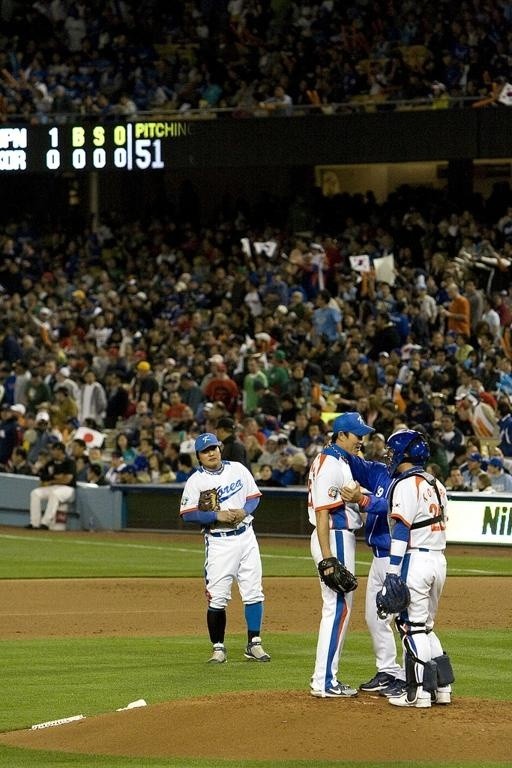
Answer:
[198,488,220,511]
[376,573,410,620]
[318,557,358,595]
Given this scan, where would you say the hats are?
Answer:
[466,452,503,485]
[1,401,51,423]
[195,433,221,452]
[214,418,235,429]
[334,413,376,437]
[286,454,307,468]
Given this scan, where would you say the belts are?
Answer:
[210,526,246,537]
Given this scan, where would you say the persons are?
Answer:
[25,442,77,530]
[1,1,512,497]
[308,412,376,699]
[180,433,271,665]
[377,430,455,708]
[324,443,408,697]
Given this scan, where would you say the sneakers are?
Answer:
[244,637,270,661]
[207,646,228,664]
[362,672,452,707]
[309,679,359,698]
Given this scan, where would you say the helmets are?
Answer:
[383,430,431,479]
